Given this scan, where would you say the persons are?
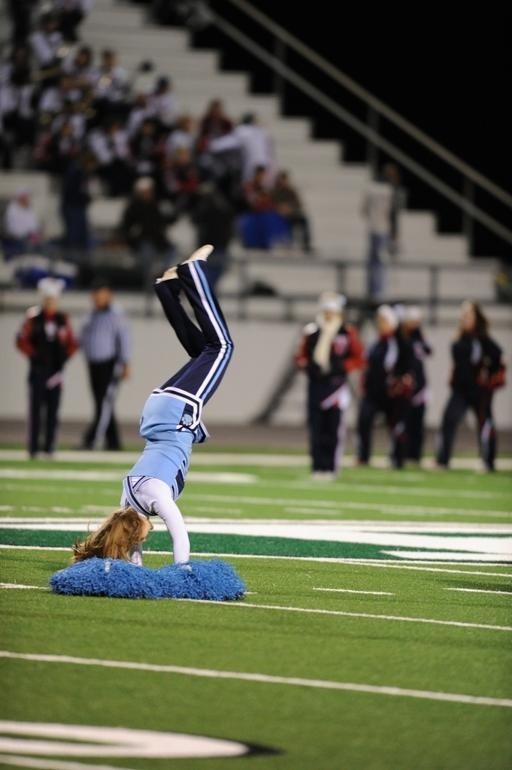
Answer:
[297,297,505,481]
[72,245,233,572]
[0,0,311,297]
[19,276,80,459]
[359,171,397,298]
[76,287,130,450]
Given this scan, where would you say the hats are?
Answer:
[317,291,421,331]
[91,275,115,288]
[38,278,65,298]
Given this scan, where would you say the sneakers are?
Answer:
[155,244,215,284]
